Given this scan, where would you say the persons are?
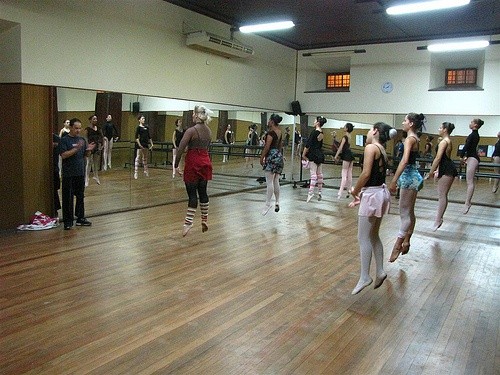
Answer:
[84,114,118,187]
[424,135,434,179]
[180,105,213,237]
[260,113,284,215]
[397,141,403,159]
[334,123,355,199]
[491,132,500,193]
[222,124,235,163]
[389,112,426,263]
[457,118,484,214]
[58,118,95,230]
[348,122,397,295]
[332,141,339,152]
[133,115,153,179]
[59,119,70,178]
[244,124,267,168]
[283,126,302,157]
[302,116,327,203]
[172,119,186,178]
[424,122,458,231]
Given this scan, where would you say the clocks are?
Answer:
[382,82,393,93]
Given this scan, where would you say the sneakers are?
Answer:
[64,222,73,230]
[76,217,92,226]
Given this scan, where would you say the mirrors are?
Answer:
[57,88,500,221]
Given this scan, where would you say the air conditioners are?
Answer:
[186,31,255,57]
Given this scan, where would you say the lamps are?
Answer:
[302,49,366,57]
